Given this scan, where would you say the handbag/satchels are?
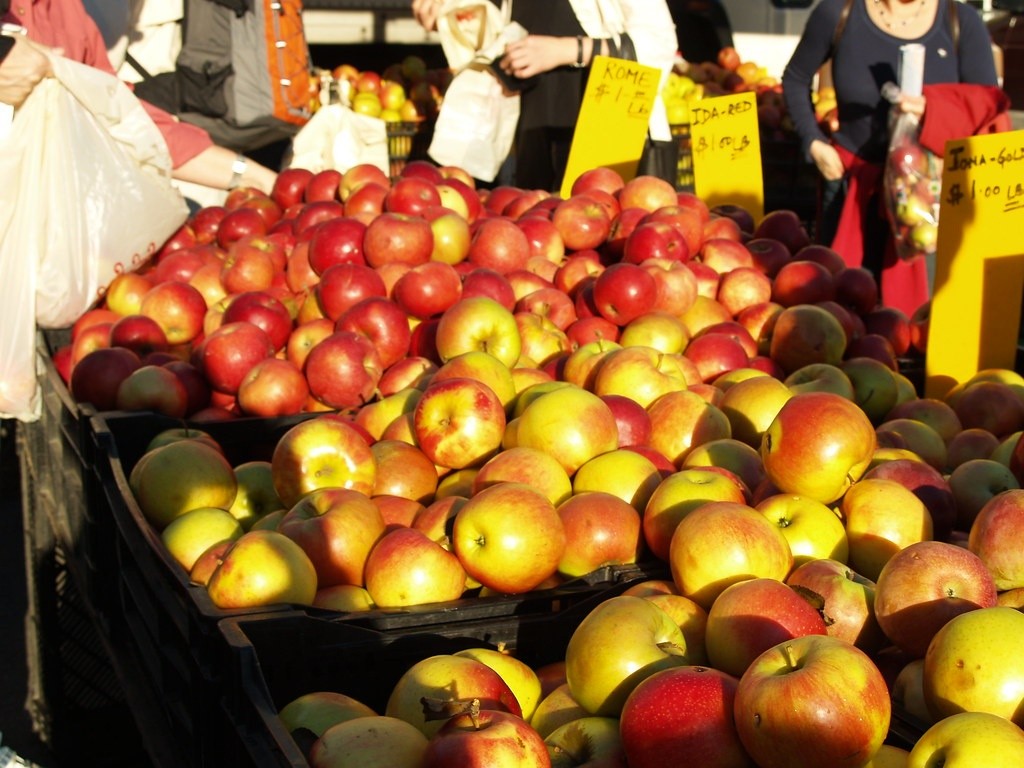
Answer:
[0,20,193,422]
[224,0,314,127]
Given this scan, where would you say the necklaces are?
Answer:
[876,0,925,30]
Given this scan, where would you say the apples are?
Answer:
[304,57,453,172]
[53,143,1024,768]
[670,47,839,141]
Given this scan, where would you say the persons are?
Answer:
[1,0,279,194]
[0,0,53,767]
[781,0,1000,305]
[666,0,734,64]
[411,0,679,189]
[124,0,292,172]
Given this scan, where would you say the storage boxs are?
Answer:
[14,112,693,768]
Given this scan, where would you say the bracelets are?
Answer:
[227,154,246,191]
[572,35,585,68]
